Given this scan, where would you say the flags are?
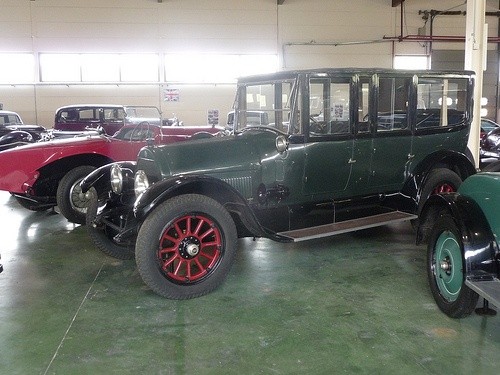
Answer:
[163,88,181,102]
